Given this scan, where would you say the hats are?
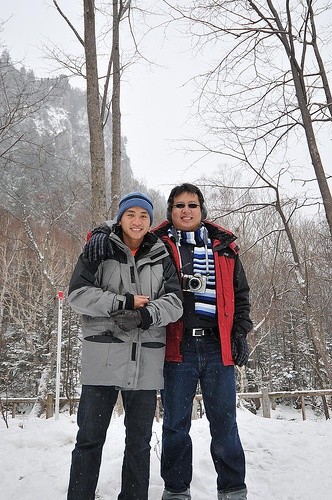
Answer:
[117,192,154,226]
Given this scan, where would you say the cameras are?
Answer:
[181,274,206,293]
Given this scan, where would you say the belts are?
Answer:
[187,327,218,336]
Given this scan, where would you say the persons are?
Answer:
[87,183,254,500]
[66,193,184,500]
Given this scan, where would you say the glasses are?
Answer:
[171,203,201,209]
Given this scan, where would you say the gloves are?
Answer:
[110,308,142,332]
[232,333,248,367]
[83,226,114,263]
